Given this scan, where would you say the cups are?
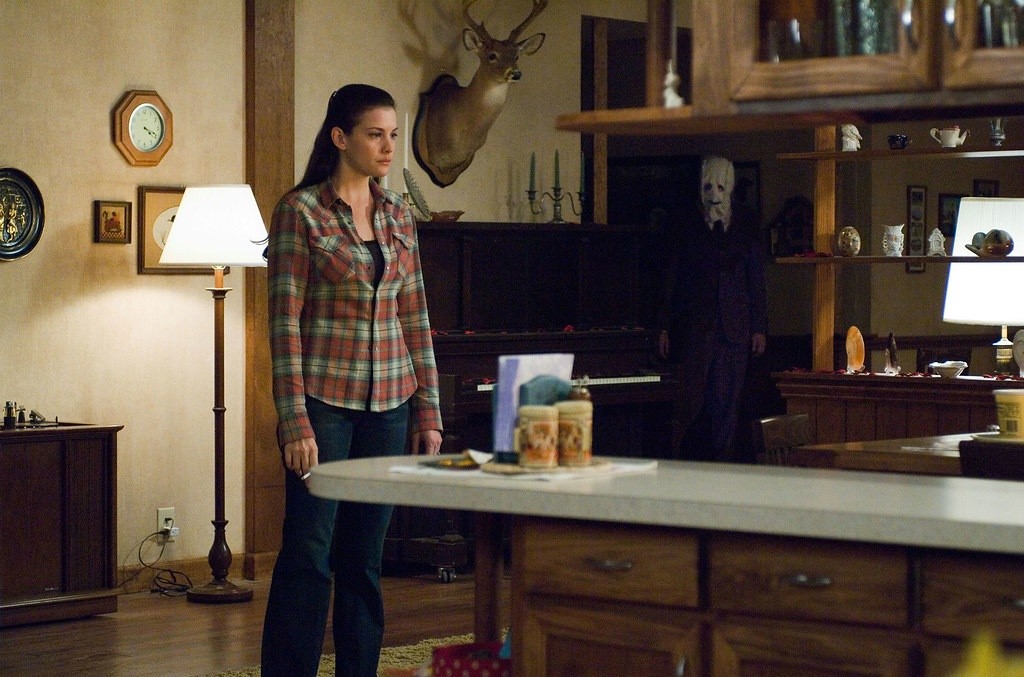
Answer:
[514,406,559,469]
[554,401,592,466]
[889,133,912,149]
[990,119,1007,147]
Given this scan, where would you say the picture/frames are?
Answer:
[974,178,1000,198]
[938,192,971,238]
[93,200,132,243]
[0,165,45,262]
[138,185,230,276]
[905,185,929,273]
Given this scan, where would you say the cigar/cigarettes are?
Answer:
[301,472,311,480]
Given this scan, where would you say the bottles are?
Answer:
[4,401,16,428]
[402,193,416,220]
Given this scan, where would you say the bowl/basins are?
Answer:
[933,366,965,378]
[431,211,465,223]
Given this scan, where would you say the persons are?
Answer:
[657,156,769,465]
[0,200,17,242]
[260,85,444,677]
[889,333,897,367]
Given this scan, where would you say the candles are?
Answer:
[554,149,560,187]
[579,151,584,192]
[529,152,535,192]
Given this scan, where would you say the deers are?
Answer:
[424,0,548,175]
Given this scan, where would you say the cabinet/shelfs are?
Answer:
[510,510,1024,676]
[557,0,1024,481]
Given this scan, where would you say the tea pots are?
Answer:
[930,126,967,147]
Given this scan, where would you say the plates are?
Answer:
[403,168,432,221]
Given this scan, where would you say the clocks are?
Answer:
[114,91,173,165]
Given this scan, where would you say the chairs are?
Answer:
[760,414,812,468]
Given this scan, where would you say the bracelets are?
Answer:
[661,330,668,334]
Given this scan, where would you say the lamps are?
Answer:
[157,507,176,543]
[157,184,272,606]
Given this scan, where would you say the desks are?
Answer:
[309,457,1024,677]
[0,421,125,629]
[796,429,998,478]
